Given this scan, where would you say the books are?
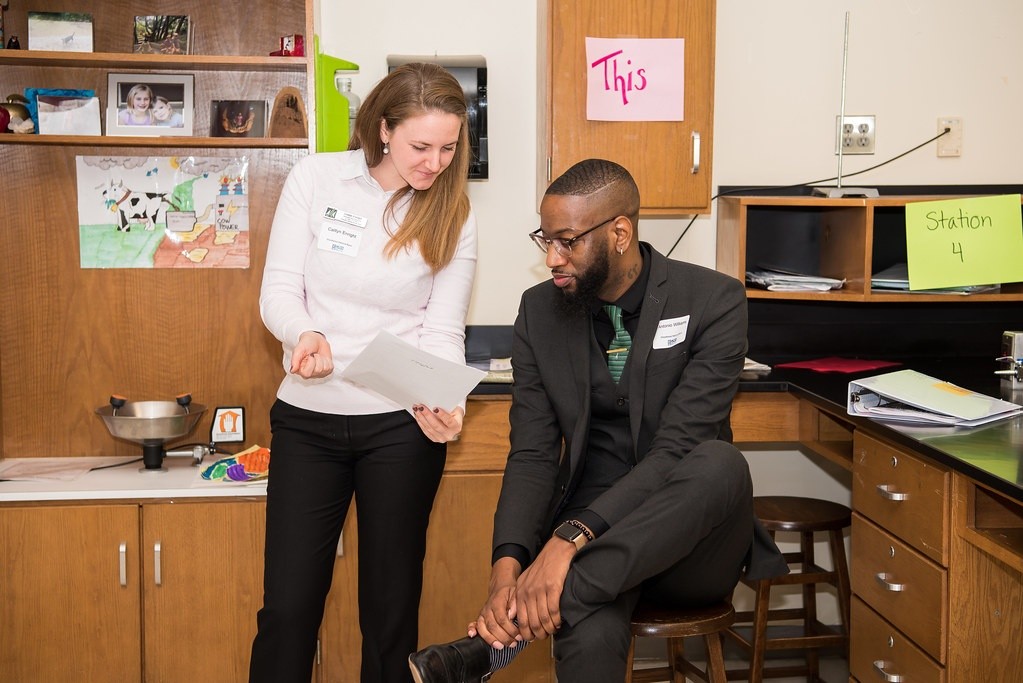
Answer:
[870,279,1001,295]
[745,268,846,291]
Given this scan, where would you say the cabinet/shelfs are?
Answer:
[318,367,799,683]
[0,0,318,683]
[716,193,1023,395]
[534,0,716,219]
[786,365,1023,683]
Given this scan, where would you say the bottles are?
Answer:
[336,78,360,136]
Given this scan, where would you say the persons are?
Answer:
[136,30,152,55]
[409,153,790,683]
[249,61,478,683]
[149,96,182,127]
[118,84,154,126]
[160,33,180,55]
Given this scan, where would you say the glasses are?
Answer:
[529,214,621,258]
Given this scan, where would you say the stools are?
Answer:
[705,496,853,683]
[624,603,736,683]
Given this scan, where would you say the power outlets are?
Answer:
[835,116,876,156]
[936,116,963,157]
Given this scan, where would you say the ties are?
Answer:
[603,305,632,384]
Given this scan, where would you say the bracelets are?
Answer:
[565,520,593,541]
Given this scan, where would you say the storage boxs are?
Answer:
[130,16,195,55]
[269,33,304,58]
[208,98,268,138]
[35,93,102,136]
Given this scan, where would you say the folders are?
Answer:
[847,370,1023,426]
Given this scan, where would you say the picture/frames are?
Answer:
[106,71,195,136]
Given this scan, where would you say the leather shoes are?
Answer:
[408,634,492,683]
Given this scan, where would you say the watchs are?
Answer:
[553,523,585,551]
